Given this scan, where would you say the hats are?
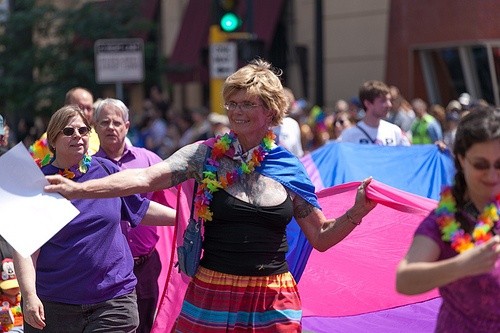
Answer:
[0,258,20,289]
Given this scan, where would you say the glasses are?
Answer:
[464,154,500,170]
[224,102,265,111]
[59,127,89,136]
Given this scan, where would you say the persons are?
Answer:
[44,59,378,333]
[0,81,500,333]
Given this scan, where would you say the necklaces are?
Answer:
[193,129,276,222]
[36,152,92,179]
[437,186,500,254]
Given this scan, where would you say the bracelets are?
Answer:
[346,209,363,226]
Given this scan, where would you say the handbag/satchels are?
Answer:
[177,219,203,277]
